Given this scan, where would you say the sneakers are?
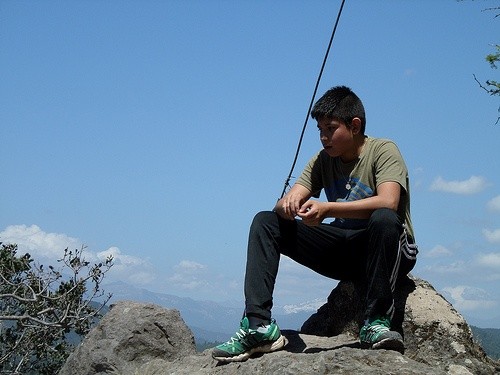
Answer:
[212,316,284,362]
[358,313,405,354]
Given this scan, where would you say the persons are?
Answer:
[210,85,420,363]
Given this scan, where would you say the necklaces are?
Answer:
[336,137,368,190]
